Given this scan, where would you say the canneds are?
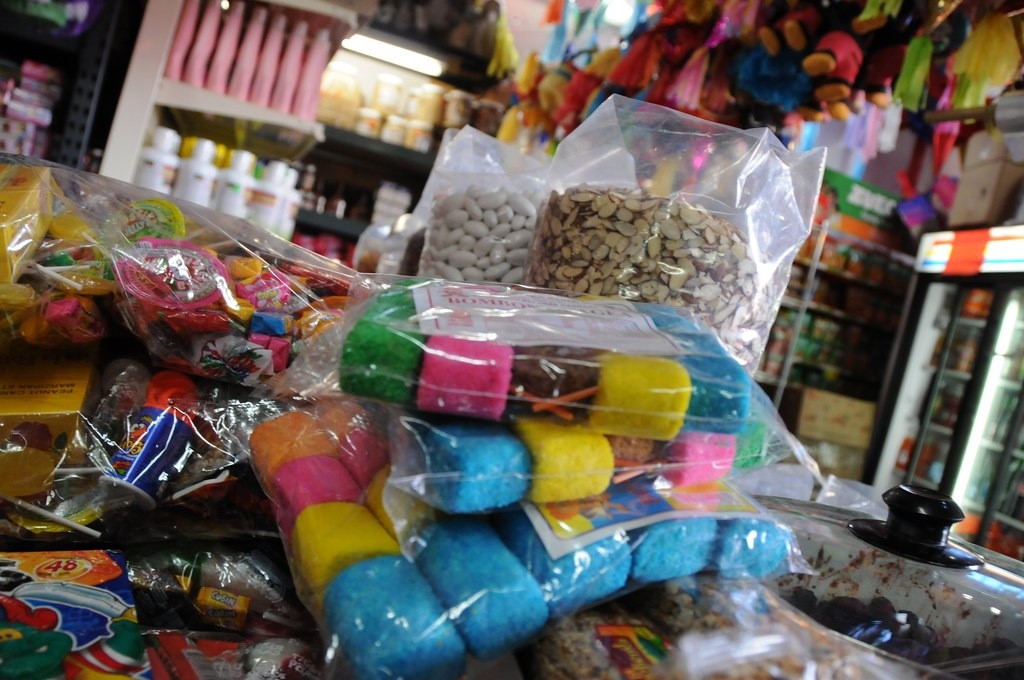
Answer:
[357,74,468,154]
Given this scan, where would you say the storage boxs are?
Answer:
[784,385,875,486]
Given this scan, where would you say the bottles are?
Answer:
[135,127,181,194]
[172,139,218,207]
[277,168,303,242]
[249,160,289,234]
[211,150,255,219]
[896,288,1024,520]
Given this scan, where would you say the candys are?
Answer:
[0,164,367,680]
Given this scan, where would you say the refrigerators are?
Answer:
[859,219,1024,563]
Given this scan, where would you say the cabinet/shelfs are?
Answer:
[280,25,509,276]
[98,0,380,243]
[750,165,921,462]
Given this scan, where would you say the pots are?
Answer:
[749,484,1024,680]
